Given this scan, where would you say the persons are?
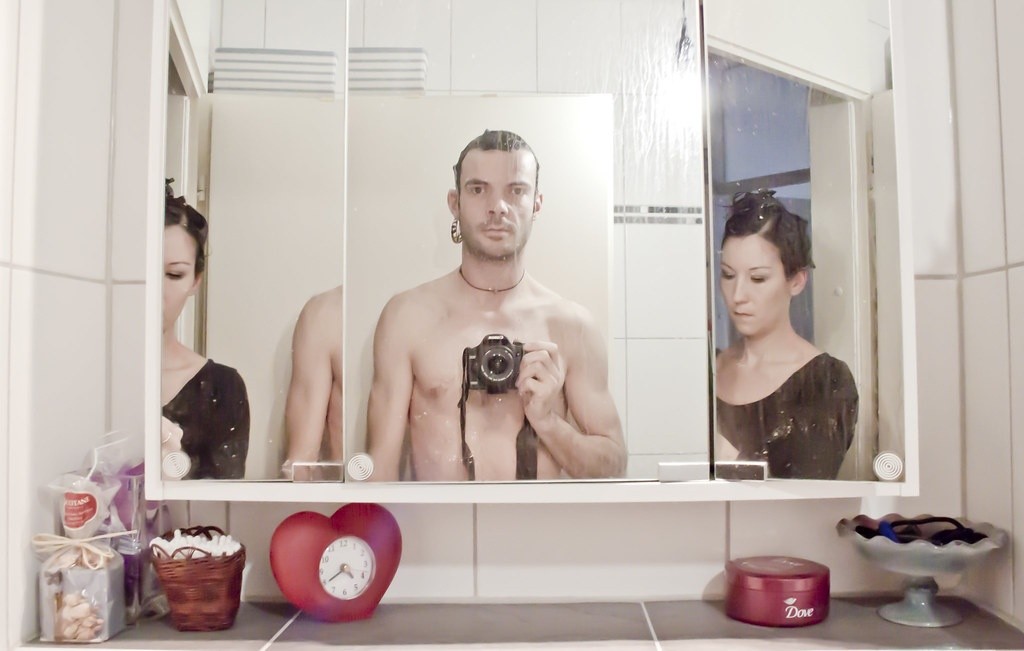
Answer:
[163,176,252,482]
[282,283,344,482]
[362,128,629,481]
[715,187,860,480]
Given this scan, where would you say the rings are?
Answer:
[162,432,171,443]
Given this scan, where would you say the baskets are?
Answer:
[151,525,245,631]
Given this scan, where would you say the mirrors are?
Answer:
[155,0,909,490]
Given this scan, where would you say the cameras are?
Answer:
[463,334,529,395]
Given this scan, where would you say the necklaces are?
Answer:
[459,265,525,293]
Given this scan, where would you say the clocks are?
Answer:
[269,502,403,622]
